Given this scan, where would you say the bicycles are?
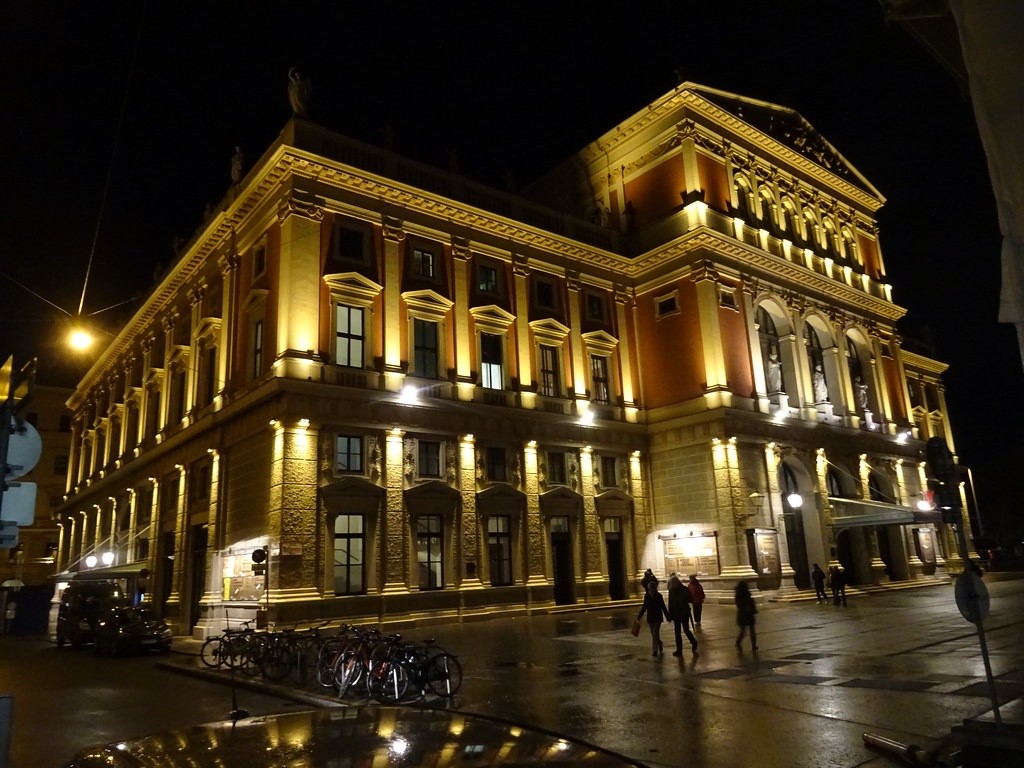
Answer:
[200,612,465,703]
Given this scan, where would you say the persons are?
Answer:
[826,566,847,607]
[636,569,705,657]
[811,563,829,606]
[732,580,759,653]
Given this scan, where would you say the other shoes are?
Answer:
[735,643,742,652]
[659,642,663,651]
[752,647,758,650]
[843,605,847,607]
[673,650,682,656]
[695,622,701,626]
[692,641,698,652]
[833,602,839,606]
[652,651,657,656]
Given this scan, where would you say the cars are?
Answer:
[93,602,175,657]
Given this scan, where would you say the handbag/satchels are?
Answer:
[631,620,640,637]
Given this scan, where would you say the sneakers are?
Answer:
[816,601,820,605]
[825,601,828,605]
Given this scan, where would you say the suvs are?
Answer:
[979,549,1012,572]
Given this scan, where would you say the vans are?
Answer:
[56,586,127,645]
